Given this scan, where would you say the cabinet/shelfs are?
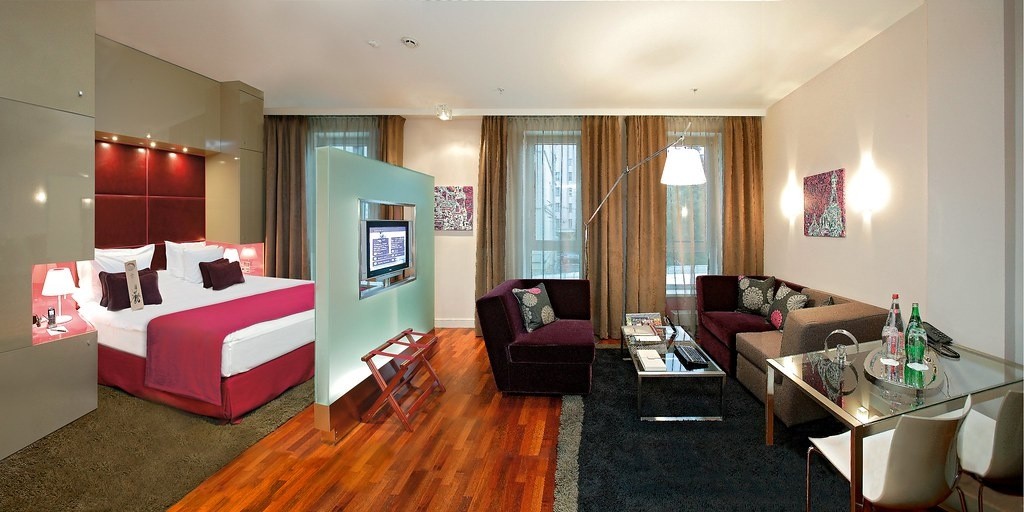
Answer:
[361,328,446,432]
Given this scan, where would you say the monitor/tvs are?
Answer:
[364,220,413,281]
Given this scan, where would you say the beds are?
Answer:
[71,269,315,425]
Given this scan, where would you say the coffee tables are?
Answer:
[620,325,726,422]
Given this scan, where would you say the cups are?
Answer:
[880,358,900,382]
[907,362,929,388]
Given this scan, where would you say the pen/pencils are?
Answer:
[52,330,66,332]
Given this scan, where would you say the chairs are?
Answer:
[936,390,1024,512]
[806,394,972,512]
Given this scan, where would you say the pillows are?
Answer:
[75,240,245,312]
[735,274,775,317]
[819,296,834,307]
[764,281,811,334]
[511,282,558,333]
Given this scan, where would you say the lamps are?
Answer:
[435,104,452,120]
[32,263,58,311]
[583,122,707,280]
[223,248,240,264]
[240,247,257,272]
[41,267,76,323]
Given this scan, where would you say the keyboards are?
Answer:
[674,344,709,368]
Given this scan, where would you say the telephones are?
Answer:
[921,321,953,345]
[47,308,56,328]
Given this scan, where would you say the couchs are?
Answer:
[476,279,595,395]
[695,275,890,424]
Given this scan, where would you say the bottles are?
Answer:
[881,294,905,383]
[904,303,928,387]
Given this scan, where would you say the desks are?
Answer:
[765,335,1024,512]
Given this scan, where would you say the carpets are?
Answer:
[0,376,315,512]
[553,344,947,512]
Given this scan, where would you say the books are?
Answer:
[622,324,666,372]
